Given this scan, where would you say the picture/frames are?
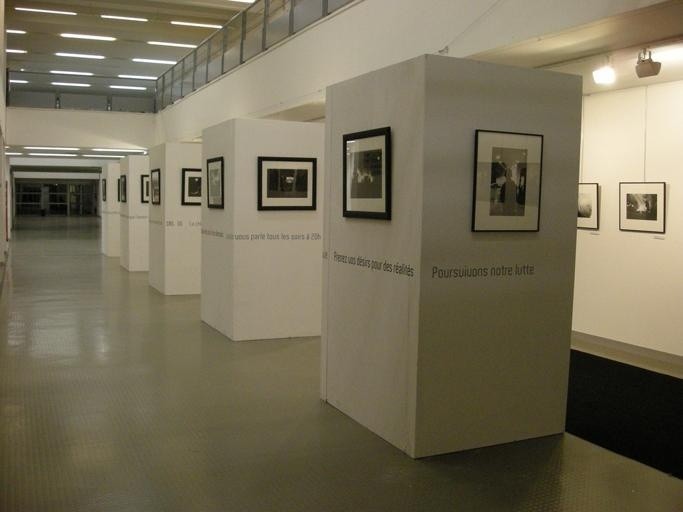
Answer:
[471,128,545,233]
[150,168,160,205]
[206,157,224,210]
[181,168,202,206]
[256,156,317,210]
[341,126,392,221]
[118,179,120,201]
[120,175,126,202]
[618,181,665,235]
[103,179,106,202]
[577,182,600,228]
[140,175,150,203]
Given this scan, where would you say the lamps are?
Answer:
[635,47,662,78]
